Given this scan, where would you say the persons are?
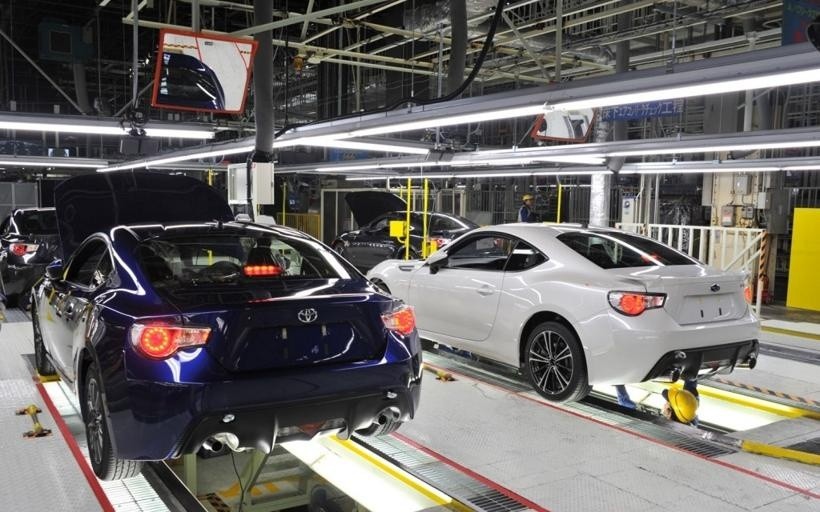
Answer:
[615,378,701,428]
[517,194,534,222]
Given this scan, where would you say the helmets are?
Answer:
[661,387,699,424]
[523,194,533,201]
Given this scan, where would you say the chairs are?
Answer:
[588,243,615,268]
[135,249,288,272]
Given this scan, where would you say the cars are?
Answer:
[0,206,64,309]
[331,193,480,275]
[26,167,424,481]
[157,52,225,110]
[537,111,590,139]
[365,221,760,404]
